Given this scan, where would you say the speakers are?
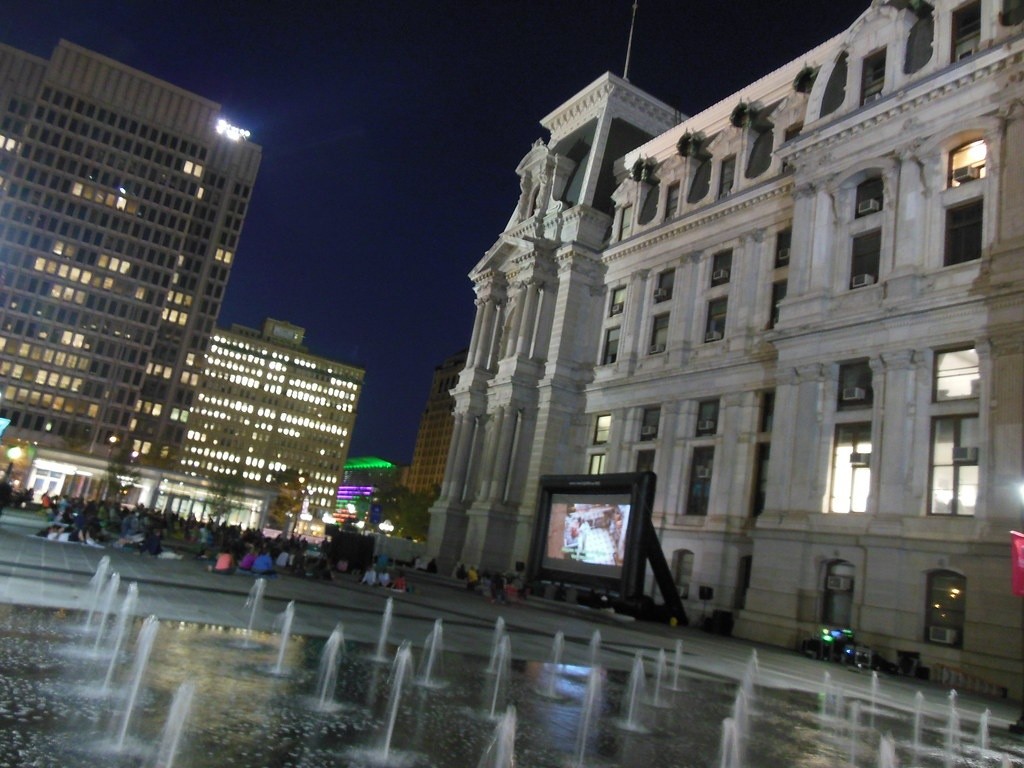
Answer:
[515,561,526,571]
[698,586,714,600]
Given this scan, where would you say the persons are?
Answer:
[37,493,533,604]
[13,488,34,510]
[514,174,532,222]
[565,503,624,566]
[536,167,553,210]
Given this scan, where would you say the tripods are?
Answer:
[694,600,708,628]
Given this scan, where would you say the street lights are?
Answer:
[3,446,23,482]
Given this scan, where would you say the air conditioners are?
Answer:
[675,585,689,598]
[642,426,656,435]
[778,248,790,261]
[827,575,852,591]
[706,330,722,342]
[713,269,728,280]
[612,304,623,314]
[958,49,978,61]
[697,468,712,479]
[858,199,879,214]
[929,625,957,645]
[851,452,870,468]
[853,274,875,288]
[653,288,667,298]
[864,93,881,106]
[698,420,714,430]
[842,387,866,401]
[951,446,978,465]
[649,344,665,355]
[954,166,979,184]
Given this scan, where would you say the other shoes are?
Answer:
[134,551,141,557]
[82,541,89,547]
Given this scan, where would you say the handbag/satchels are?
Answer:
[406,582,414,593]
